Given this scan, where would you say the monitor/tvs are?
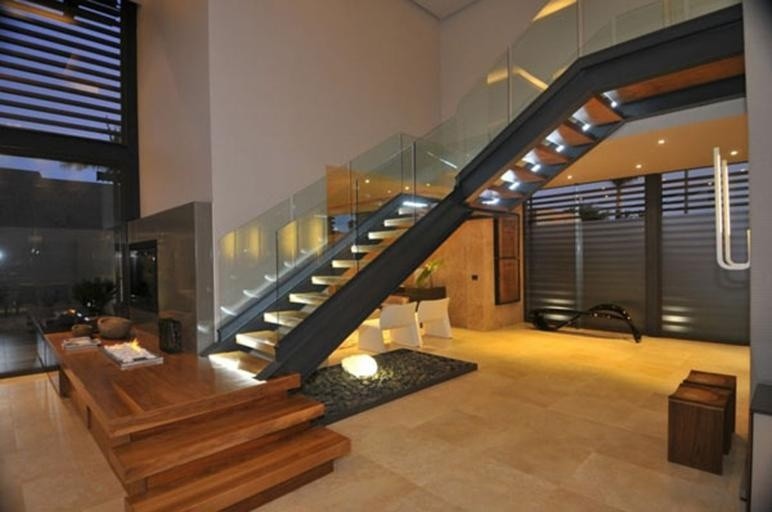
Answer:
[128,239,159,316]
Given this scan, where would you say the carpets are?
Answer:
[297,348,478,426]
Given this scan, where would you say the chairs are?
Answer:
[357,298,453,353]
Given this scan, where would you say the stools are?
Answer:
[667,368,736,476]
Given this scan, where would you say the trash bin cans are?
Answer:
[159,318,182,355]
